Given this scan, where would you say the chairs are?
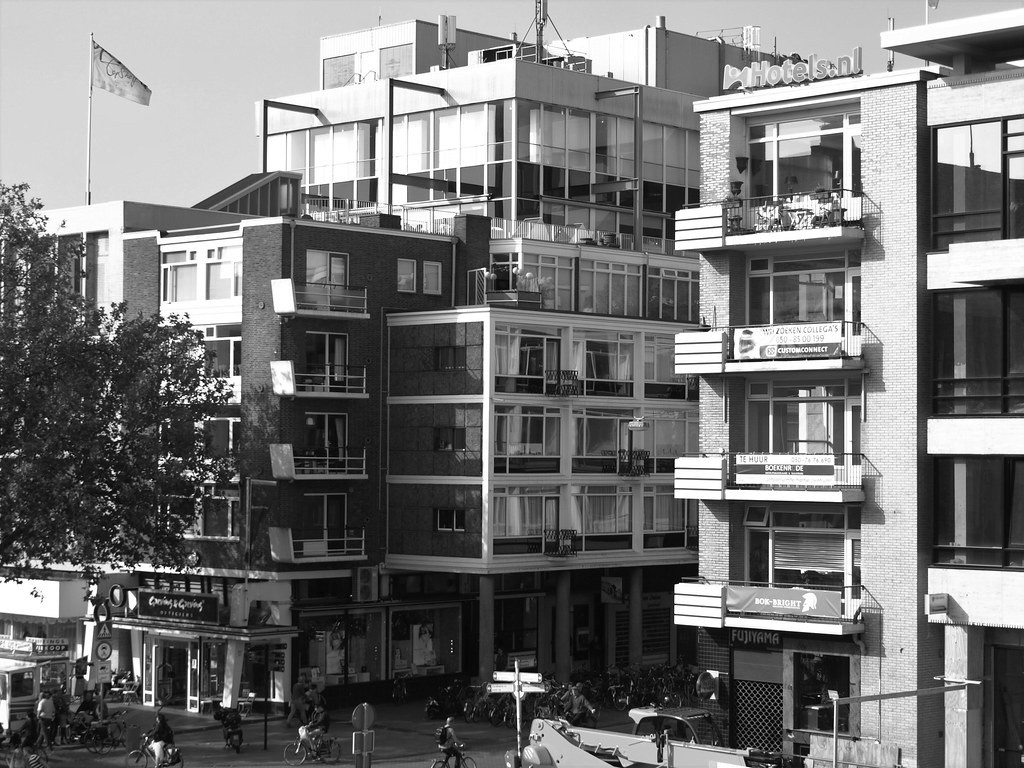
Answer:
[754,200,784,232]
[110,678,140,705]
[811,191,840,228]
[238,689,256,718]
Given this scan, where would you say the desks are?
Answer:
[782,207,812,230]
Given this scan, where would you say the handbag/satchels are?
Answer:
[299,726,311,742]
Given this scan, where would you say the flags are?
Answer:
[92,42,152,109]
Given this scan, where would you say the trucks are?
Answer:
[0,657,74,736]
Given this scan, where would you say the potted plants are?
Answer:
[311,447,329,474]
[724,195,743,208]
[312,368,327,393]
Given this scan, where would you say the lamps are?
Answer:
[629,416,651,431]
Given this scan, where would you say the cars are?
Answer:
[627,707,725,746]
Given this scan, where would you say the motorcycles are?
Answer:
[215,712,245,754]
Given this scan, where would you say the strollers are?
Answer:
[302,696,328,724]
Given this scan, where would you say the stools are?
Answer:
[729,214,742,232]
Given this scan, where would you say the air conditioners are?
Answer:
[351,567,379,601]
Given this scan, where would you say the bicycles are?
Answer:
[429,741,480,768]
[129,733,185,768]
[0,731,50,765]
[84,708,134,755]
[282,736,343,765]
[574,654,697,712]
[390,672,415,705]
[426,679,599,730]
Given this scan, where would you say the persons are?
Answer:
[287,676,329,755]
[0,683,109,768]
[418,624,433,653]
[327,632,345,674]
[145,713,173,768]
[590,635,602,667]
[496,647,506,670]
[561,682,596,727]
[439,716,465,768]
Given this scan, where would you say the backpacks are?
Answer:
[435,726,453,744]
